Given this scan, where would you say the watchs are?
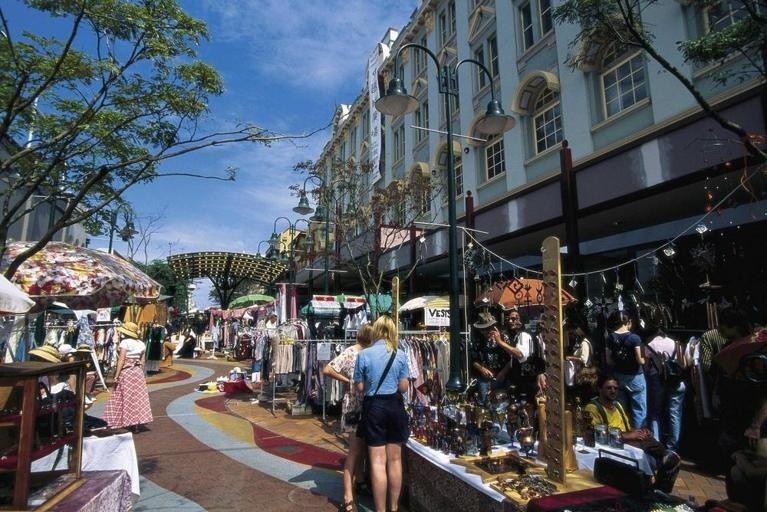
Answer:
[733,454,741,462]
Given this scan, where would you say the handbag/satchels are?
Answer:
[515,330,546,378]
[356,346,397,438]
[573,340,598,387]
[342,409,361,428]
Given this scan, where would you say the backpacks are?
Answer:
[647,338,683,388]
[649,342,683,385]
[612,329,635,371]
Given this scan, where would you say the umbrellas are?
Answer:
[229,294,275,306]
[473,278,580,312]
[399,296,440,311]
[0,239,164,362]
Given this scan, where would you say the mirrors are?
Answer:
[0,471,131,512]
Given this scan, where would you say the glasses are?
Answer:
[545,445,561,454]
[542,337,559,346]
[545,396,560,404]
[536,304,558,312]
[543,373,559,382]
[545,362,560,370]
[543,408,560,417]
[542,349,559,358]
[540,269,558,279]
[540,314,558,322]
[540,292,557,299]
[540,325,559,335]
[506,317,519,322]
[544,455,561,464]
[546,431,562,444]
[543,466,561,477]
[541,281,557,289]
[544,384,561,394]
[544,419,560,428]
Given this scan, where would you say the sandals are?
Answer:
[343,501,357,512]
[353,480,371,496]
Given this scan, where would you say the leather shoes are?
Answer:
[129,425,140,435]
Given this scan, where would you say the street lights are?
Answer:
[268,216,317,321]
[20,190,74,241]
[254,239,290,297]
[293,175,359,297]
[88,202,139,254]
[375,43,517,393]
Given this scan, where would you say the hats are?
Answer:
[58,344,77,353]
[473,313,496,328]
[564,312,588,327]
[116,321,139,339]
[78,343,92,353]
[28,345,62,363]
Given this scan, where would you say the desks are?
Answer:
[502,434,678,477]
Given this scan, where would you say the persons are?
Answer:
[605,312,647,433]
[744,396,765,453]
[57,343,76,395]
[161,336,178,364]
[321,321,371,511]
[491,308,545,408]
[729,418,766,511]
[642,324,685,453]
[582,377,682,501]
[102,321,153,432]
[468,312,510,402]
[709,306,766,452]
[353,314,410,512]
[73,345,97,405]
[268,314,278,328]
[4,347,62,438]
[565,327,598,406]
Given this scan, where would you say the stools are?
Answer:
[0,361,88,505]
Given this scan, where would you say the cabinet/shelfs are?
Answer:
[405,435,640,512]
